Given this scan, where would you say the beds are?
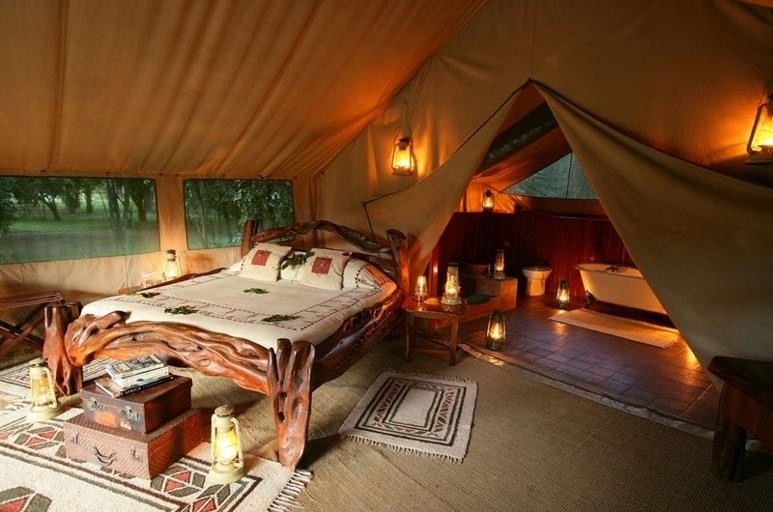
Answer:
[41,221,422,468]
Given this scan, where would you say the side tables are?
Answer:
[400,295,466,365]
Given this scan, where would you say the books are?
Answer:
[95,354,173,398]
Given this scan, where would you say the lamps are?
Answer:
[26,363,64,422]
[480,192,495,214]
[441,261,463,313]
[484,310,507,350]
[492,248,505,280]
[390,125,417,177]
[205,403,244,483]
[554,279,571,309]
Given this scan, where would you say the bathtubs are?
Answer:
[575,262,668,315]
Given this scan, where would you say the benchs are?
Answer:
[0,289,65,362]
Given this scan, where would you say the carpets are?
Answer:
[335,366,475,465]
[547,306,679,350]
[0,353,311,512]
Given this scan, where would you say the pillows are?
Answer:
[238,240,350,292]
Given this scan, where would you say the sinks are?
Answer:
[521,266,552,296]
